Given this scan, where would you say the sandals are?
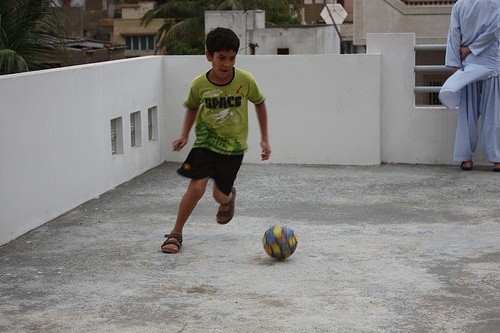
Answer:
[216,187,236,225]
[161,233,183,253]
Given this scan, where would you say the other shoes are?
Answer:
[492,163,500,172]
[460,161,474,170]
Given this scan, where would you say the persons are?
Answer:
[161,27,271,253]
[438,0,500,172]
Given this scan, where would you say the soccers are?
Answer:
[261,224,299,258]
